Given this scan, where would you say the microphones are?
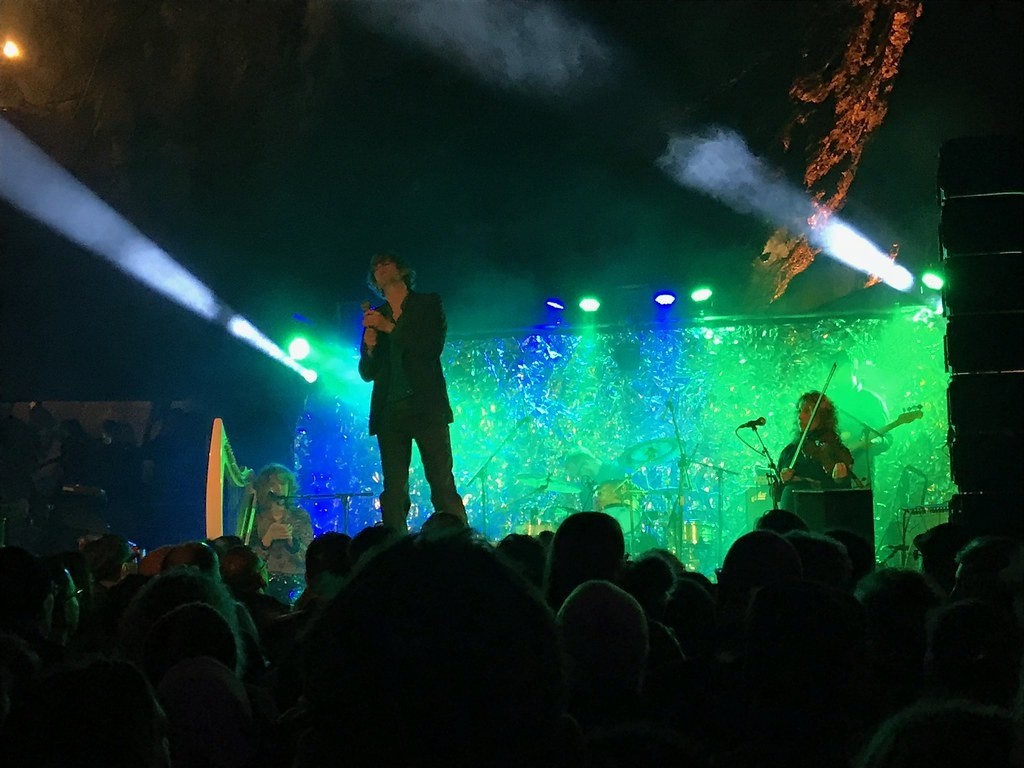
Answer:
[267,489,279,500]
[523,400,533,433]
[739,418,766,429]
[360,301,372,329]
[661,386,675,419]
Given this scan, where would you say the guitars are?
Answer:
[839,403,924,454]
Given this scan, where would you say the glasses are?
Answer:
[715,568,723,580]
[61,586,84,602]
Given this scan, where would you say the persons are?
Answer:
[234,463,316,604]
[0,512,1024,768]
[357,250,468,532]
[774,391,854,488]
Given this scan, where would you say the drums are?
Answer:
[517,520,559,538]
[591,479,643,535]
[667,520,714,548]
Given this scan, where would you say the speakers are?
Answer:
[792,488,875,571]
[886,544,909,551]
[931,134,1024,537]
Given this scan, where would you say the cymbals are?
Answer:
[539,507,580,525]
[515,474,582,494]
[646,486,689,495]
[618,438,686,467]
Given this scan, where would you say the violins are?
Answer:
[801,430,871,490]
